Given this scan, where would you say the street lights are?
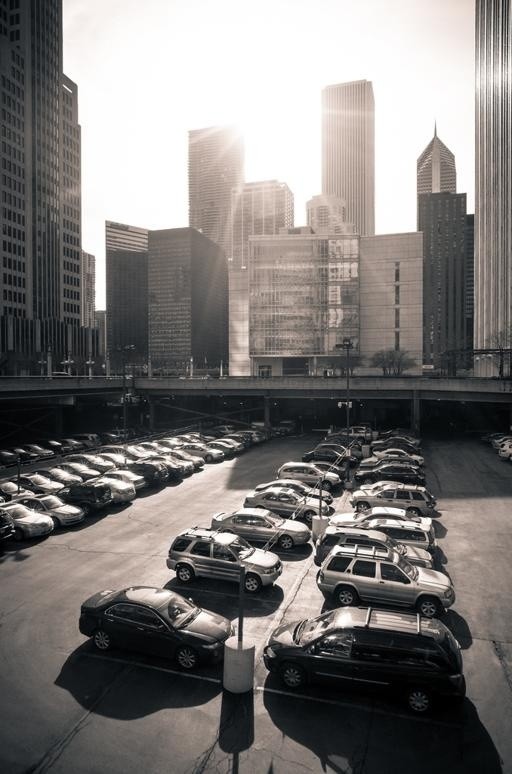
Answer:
[342,338,354,481]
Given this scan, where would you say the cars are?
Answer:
[487,431,512,461]
